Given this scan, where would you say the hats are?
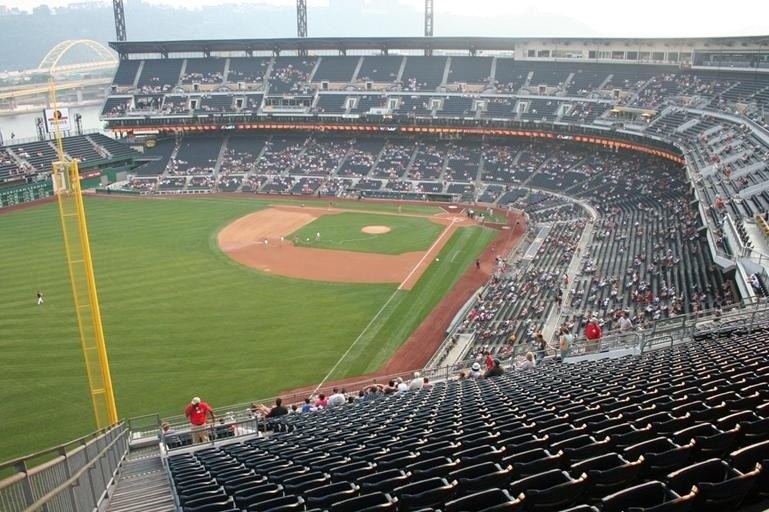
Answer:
[190,397,201,405]
[161,422,170,428]
[471,362,481,370]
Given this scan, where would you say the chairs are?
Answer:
[157,379,468,512]
[462,56,769,511]
[2,133,141,185]
[100,47,468,205]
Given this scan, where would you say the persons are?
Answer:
[248,361,435,419]
[35,290,44,304]
[0,140,108,182]
[182,397,216,444]
[217,417,234,432]
[280,232,285,245]
[327,199,334,211]
[316,229,321,240]
[263,238,270,250]
[295,233,301,246]
[161,422,182,445]
